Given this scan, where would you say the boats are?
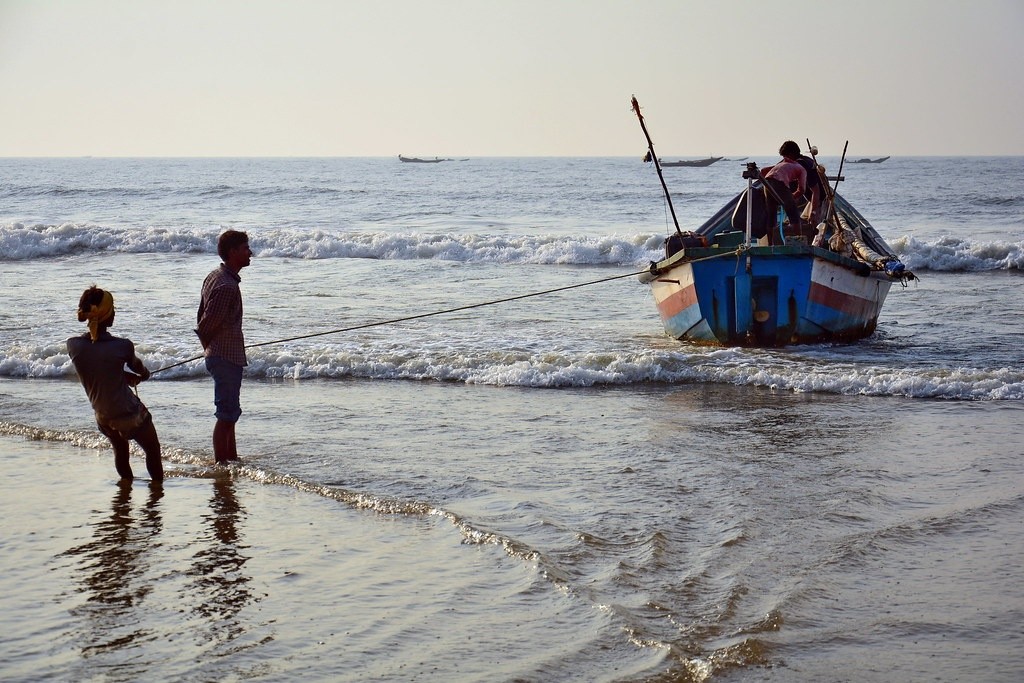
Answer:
[631,94,925,349]
[655,156,724,168]
[843,156,895,167]
[397,153,445,164]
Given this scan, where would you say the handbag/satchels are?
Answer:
[663,231,708,259]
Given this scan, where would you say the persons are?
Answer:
[754,141,820,217]
[196,232,253,463]
[68,288,164,483]
